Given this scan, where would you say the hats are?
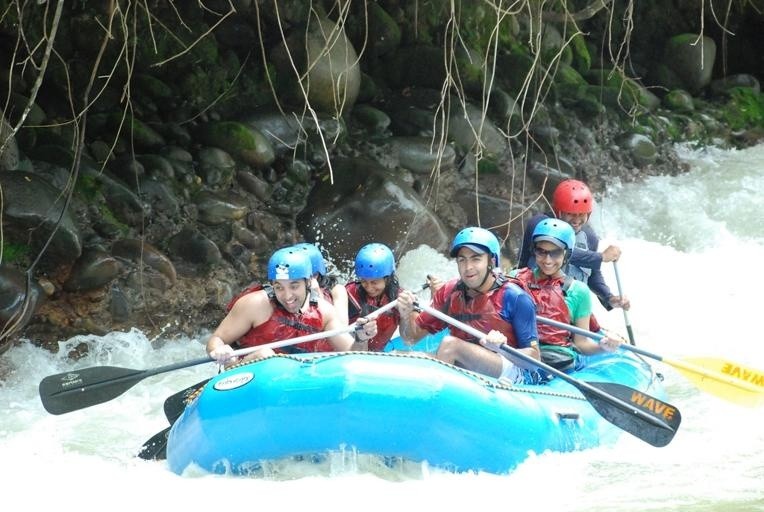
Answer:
[534,234,566,250]
[454,244,485,255]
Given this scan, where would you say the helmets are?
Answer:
[553,179,593,214]
[449,227,500,267]
[292,242,326,276]
[355,243,395,280]
[530,217,577,259]
[267,246,313,281]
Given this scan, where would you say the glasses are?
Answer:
[532,247,562,259]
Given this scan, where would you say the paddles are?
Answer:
[139,427,173,459]
[535,314,764,407]
[163,275,437,427]
[38,316,371,416]
[411,299,682,448]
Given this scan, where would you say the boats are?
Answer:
[166,329,665,475]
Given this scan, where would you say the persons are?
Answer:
[508,219,616,374]
[205,243,356,369]
[395,225,547,387]
[517,180,624,315]
[344,242,439,348]
